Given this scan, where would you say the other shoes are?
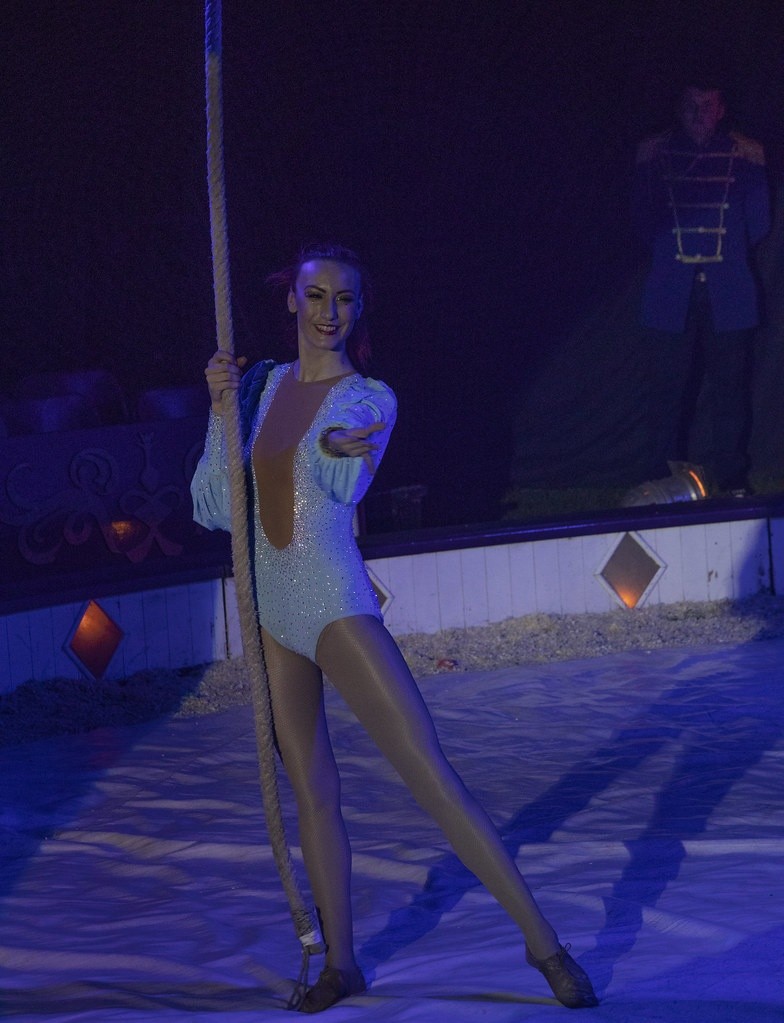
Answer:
[299,966,366,1013]
[525,940,599,1009]
[729,482,753,497]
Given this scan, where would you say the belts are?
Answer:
[694,272,707,282]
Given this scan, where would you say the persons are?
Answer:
[602,68,773,499]
[188,245,600,1013]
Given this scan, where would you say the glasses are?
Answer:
[685,101,722,114]
